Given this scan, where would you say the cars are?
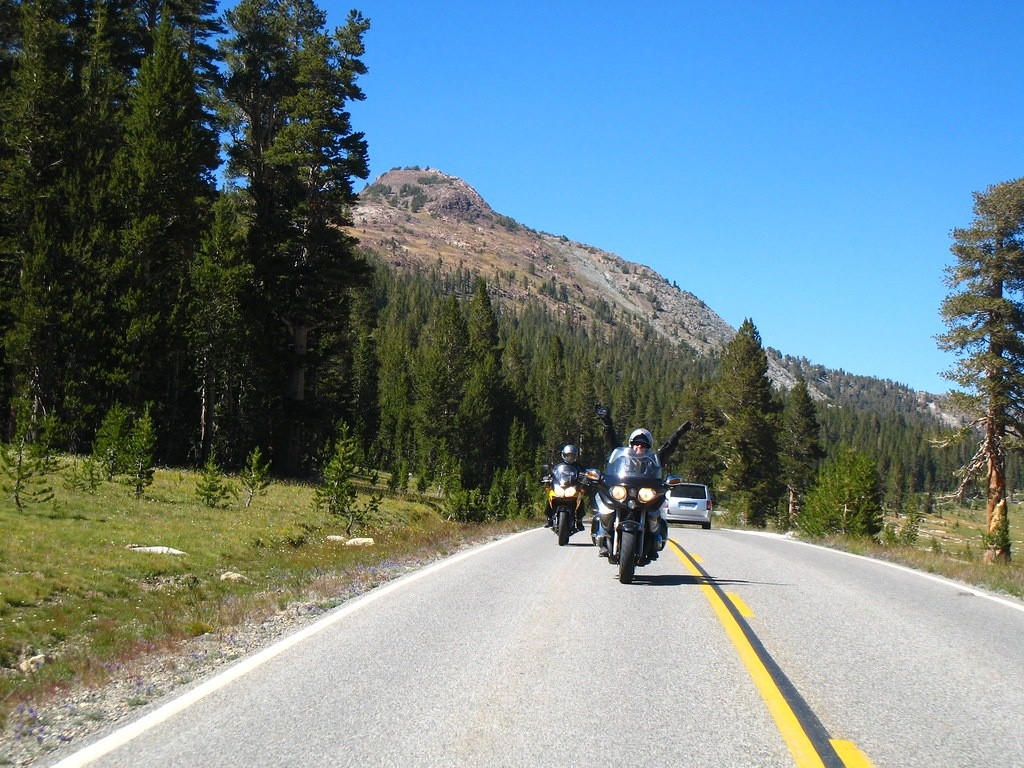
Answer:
[661,482,713,529]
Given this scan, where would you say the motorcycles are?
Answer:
[539,465,601,546]
[591,445,682,584]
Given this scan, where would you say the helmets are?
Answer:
[561,445,578,463]
[629,428,653,448]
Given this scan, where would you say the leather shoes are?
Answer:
[599,546,608,555]
[577,522,584,531]
[648,552,658,558]
[544,520,553,528]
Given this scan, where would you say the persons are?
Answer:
[596,427,663,558]
[543,445,585,531]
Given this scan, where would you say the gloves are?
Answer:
[676,420,692,437]
[543,476,548,480]
[582,478,588,484]
